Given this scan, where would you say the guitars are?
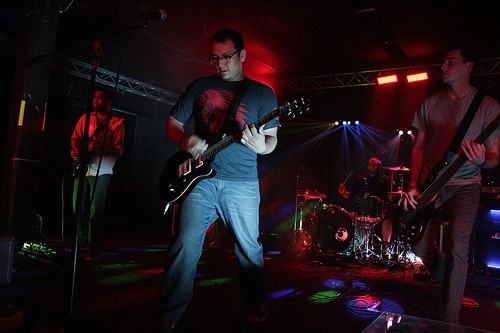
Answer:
[390,114,500,247]
[65,118,126,179]
[158,94,310,215]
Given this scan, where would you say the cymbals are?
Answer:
[383,166,410,171]
[293,192,326,199]
[387,190,406,194]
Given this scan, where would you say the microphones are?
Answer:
[143,9,168,21]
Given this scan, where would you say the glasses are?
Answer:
[207,50,238,65]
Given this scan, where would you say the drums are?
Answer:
[355,195,382,223]
[311,204,355,255]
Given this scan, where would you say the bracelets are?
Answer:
[179,134,186,148]
[476,160,487,167]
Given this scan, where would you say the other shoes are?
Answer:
[247,297,269,323]
[151,320,188,333]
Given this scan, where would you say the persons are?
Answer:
[397,48,500,324]
[338,158,388,213]
[70,88,126,261]
[150,28,280,333]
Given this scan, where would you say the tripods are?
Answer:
[352,198,406,263]
